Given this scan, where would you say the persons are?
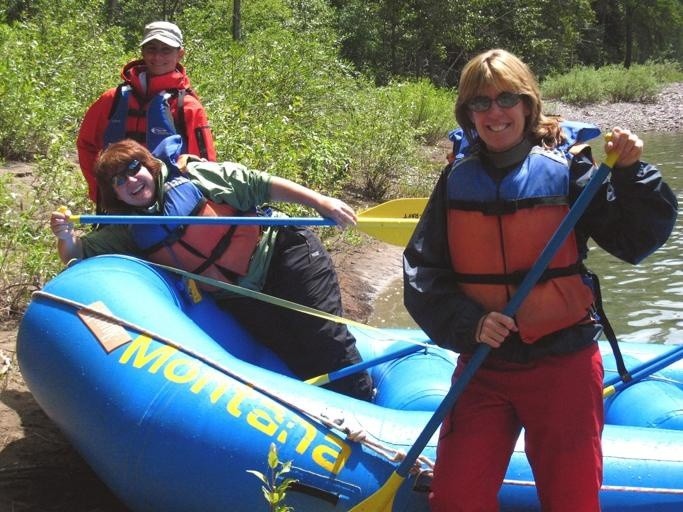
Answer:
[402,46,680,512]
[49,134,374,402]
[75,20,218,226]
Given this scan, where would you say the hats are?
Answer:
[138,21,185,50]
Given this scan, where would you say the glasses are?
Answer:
[110,158,143,188]
[463,90,528,111]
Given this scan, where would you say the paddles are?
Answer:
[348,131,619,512]
[56,198,432,246]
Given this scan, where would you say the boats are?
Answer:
[16,254,683,511]
